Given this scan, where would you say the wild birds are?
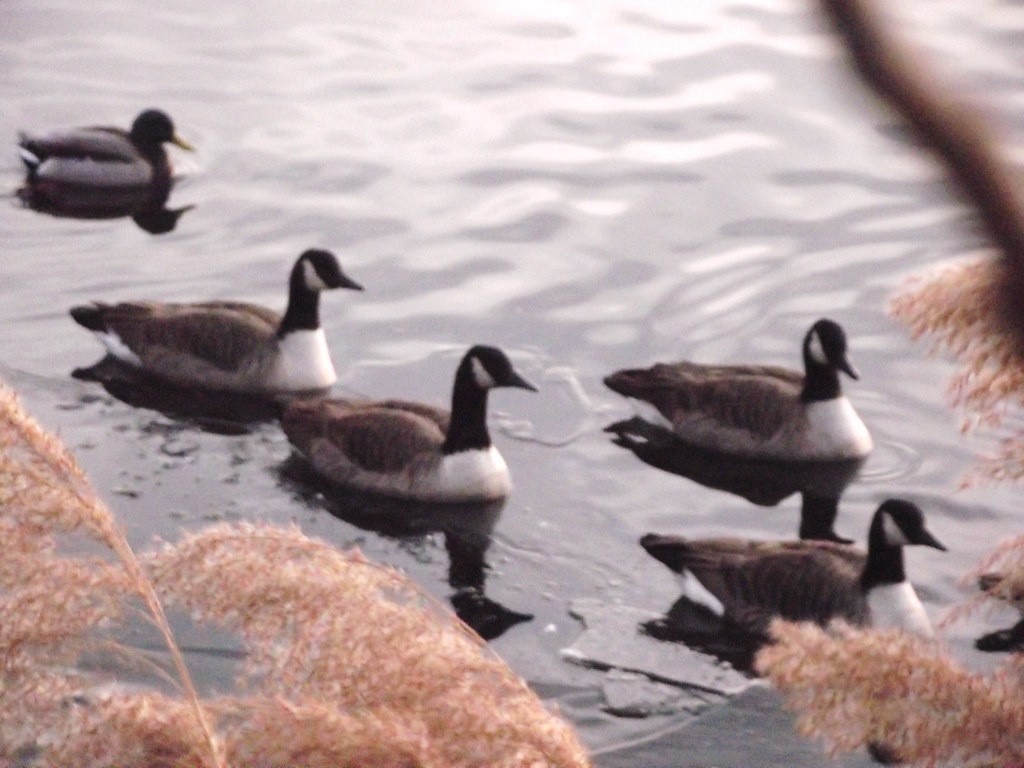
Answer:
[272,343,541,505]
[16,107,195,189]
[635,498,952,641]
[597,317,876,463]
[970,619,1024,655]
[68,249,367,395]
